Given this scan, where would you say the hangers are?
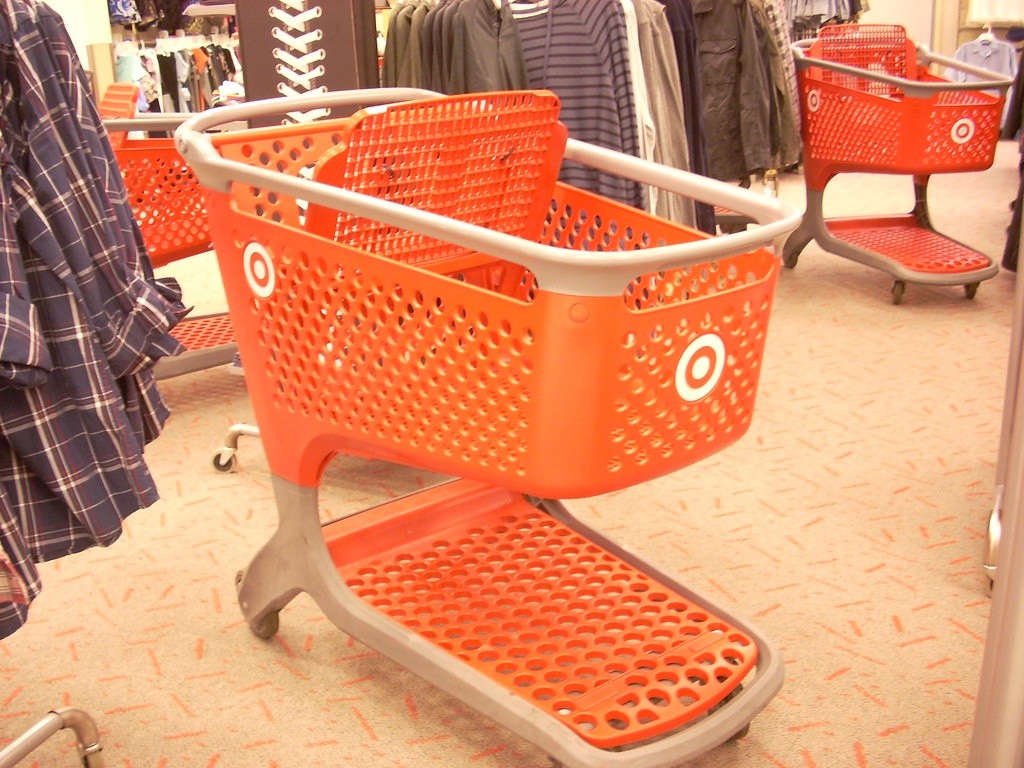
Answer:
[112,34,240,60]
[789,26,821,42]
[977,24,994,42]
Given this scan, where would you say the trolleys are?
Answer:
[780,22,1014,307]
[173,86,783,768]
[95,80,253,391]
